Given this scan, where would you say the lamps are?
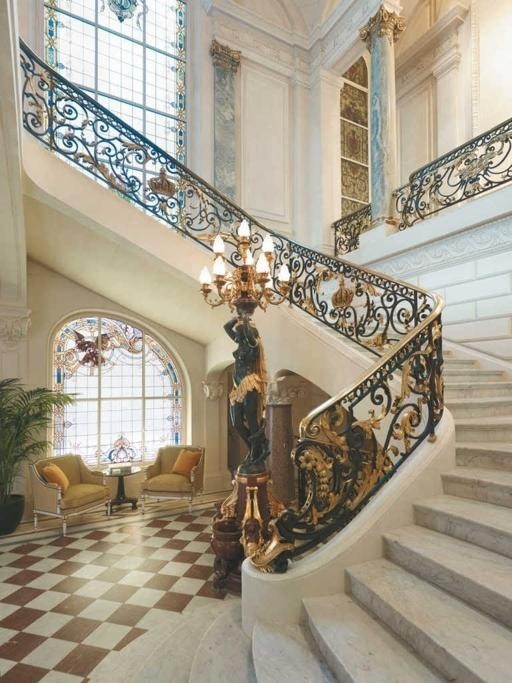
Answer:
[200,218,292,316]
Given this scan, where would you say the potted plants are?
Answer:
[0,377,77,534]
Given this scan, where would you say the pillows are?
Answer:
[42,462,70,494]
[173,449,200,477]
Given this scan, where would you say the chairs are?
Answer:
[28,456,112,536]
[141,444,205,513]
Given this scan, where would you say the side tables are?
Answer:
[100,466,143,512]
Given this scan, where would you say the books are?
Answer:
[109,463,132,474]
